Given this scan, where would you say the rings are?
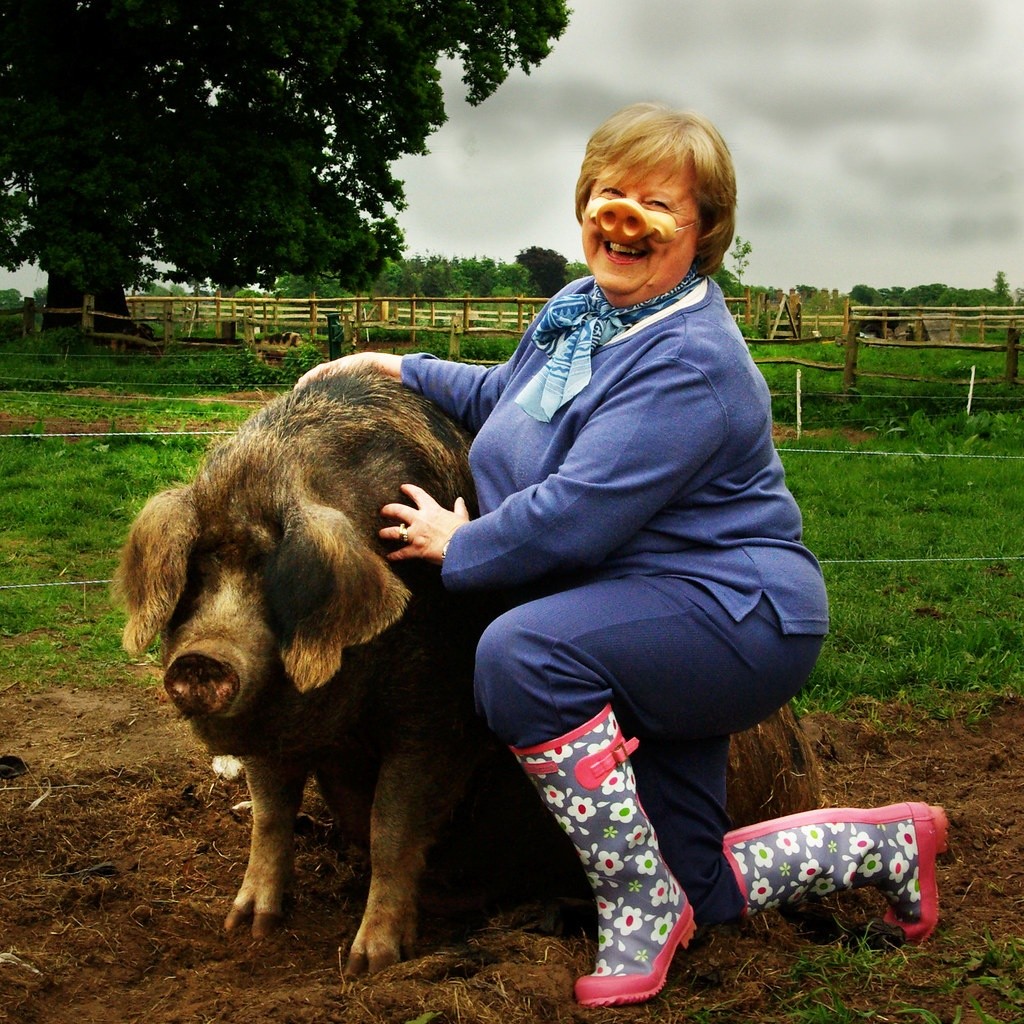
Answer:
[399,524,408,542]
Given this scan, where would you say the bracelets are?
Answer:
[442,534,452,561]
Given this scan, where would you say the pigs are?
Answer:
[105,364,822,985]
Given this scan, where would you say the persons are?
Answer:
[289,104,951,1008]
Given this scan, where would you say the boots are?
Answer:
[510,702,696,1009]
[723,801,951,944]
